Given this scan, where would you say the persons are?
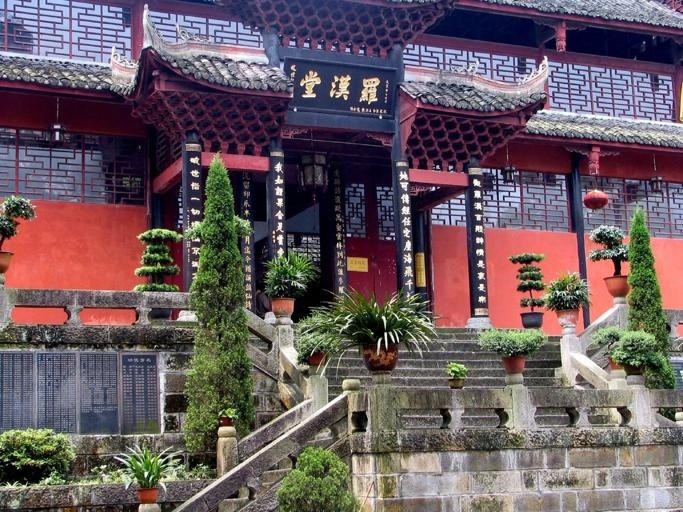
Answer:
[255,278,272,320]
